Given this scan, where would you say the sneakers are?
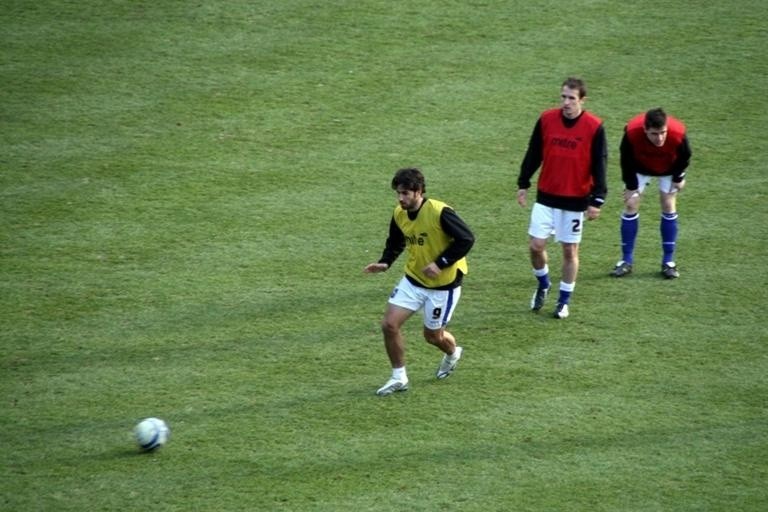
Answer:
[609,260,633,277]
[435,345,463,381]
[530,280,552,312]
[375,375,410,395]
[660,261,680,278]
[555,301,570,319]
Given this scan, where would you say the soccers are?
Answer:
[137,417,168,451]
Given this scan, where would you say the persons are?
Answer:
[364,168,475,397]
[517,77,608,319]
[608,108,691,279]
